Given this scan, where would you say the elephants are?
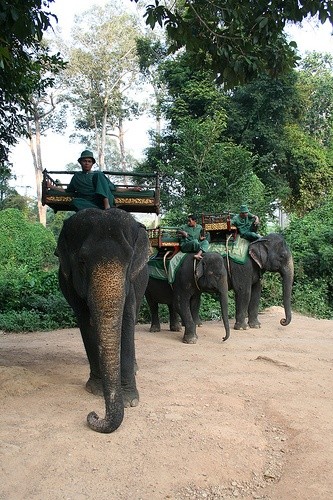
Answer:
[145,252,230,344]
[54,208,154,433]
[223,233,294,330]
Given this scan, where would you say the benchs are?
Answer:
[146,226,180,249]
[41,169,161,216]
[201,215,238,240]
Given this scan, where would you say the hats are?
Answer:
[240,205,250,213]
[78,150,96,164]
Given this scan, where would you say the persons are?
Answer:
[64,150,117,211]
[176,215,209,261]
[226,204,263,243]
